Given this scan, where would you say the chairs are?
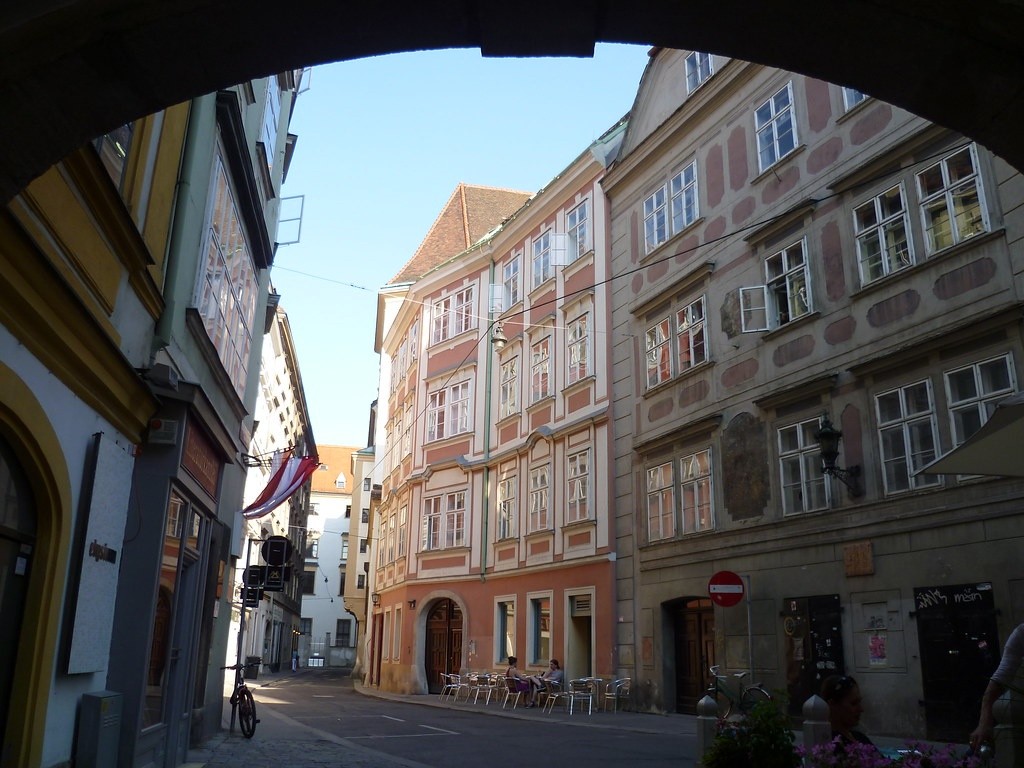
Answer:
[439,672,603,716]
[604,677,633,714]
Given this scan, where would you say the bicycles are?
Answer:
[219,661,262,739]
[704,664,774,720]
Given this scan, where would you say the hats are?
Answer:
[550,659,560,670]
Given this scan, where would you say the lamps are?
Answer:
[490,320,507,352]
[814,410,863,499]
[372,593,381,607]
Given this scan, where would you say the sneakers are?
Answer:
[536,684,546,692]
[526,701,534,709]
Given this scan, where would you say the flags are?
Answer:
[241,454,323,520]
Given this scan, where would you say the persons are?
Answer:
[525,659,563,708]
[505,656,533,708]
[292,648,298,672]
[967,623,1024,752]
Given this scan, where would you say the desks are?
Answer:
[526,675,532,701]
[590,679,602,711]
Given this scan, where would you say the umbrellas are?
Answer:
[909,391,1024,478]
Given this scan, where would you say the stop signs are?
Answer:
[707,569,745,607]
